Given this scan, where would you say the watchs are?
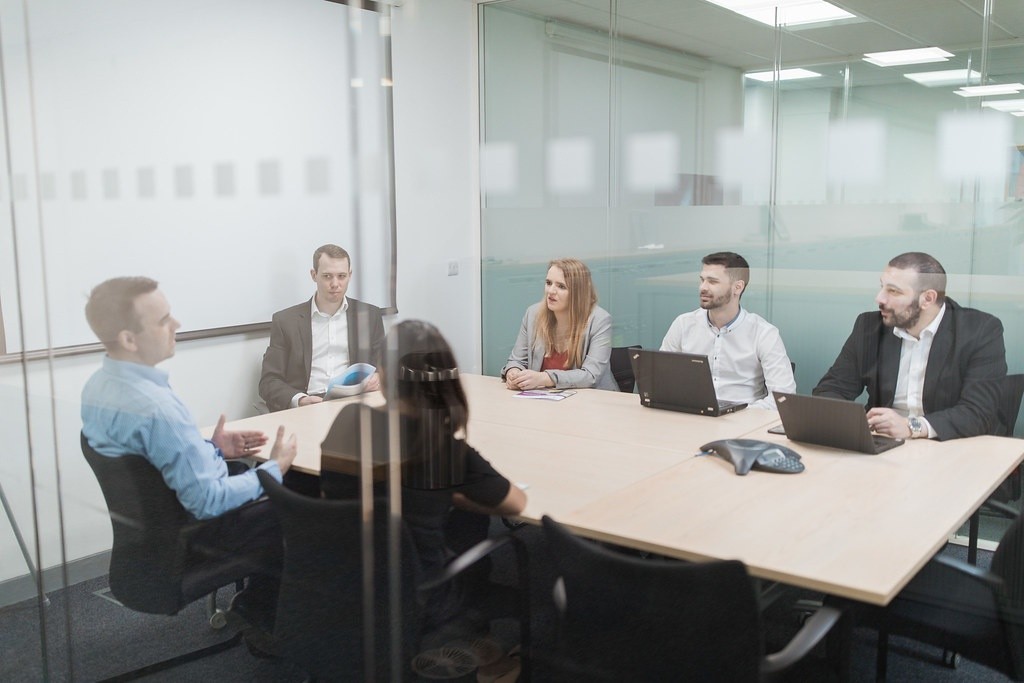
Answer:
[907,415,922,439]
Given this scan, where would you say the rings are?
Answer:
[377,384,379,386]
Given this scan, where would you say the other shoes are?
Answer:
[228,588,279,661]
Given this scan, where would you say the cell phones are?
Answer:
[768,424,786,435]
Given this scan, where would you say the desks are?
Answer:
[548,418,1024,683]
[198,373,781,683]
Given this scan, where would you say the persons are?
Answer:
[318,319,528,683]
[500,258,621,392]
[258,244,385,411]
[80,276,298,627]
[811,252,1008,442]
[659,252,797,411]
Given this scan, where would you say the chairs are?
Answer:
[80,343,1024,683]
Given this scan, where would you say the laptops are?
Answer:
[773,390,905,454]
[629,348,748,417]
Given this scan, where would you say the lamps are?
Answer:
[546,22,714,79]
